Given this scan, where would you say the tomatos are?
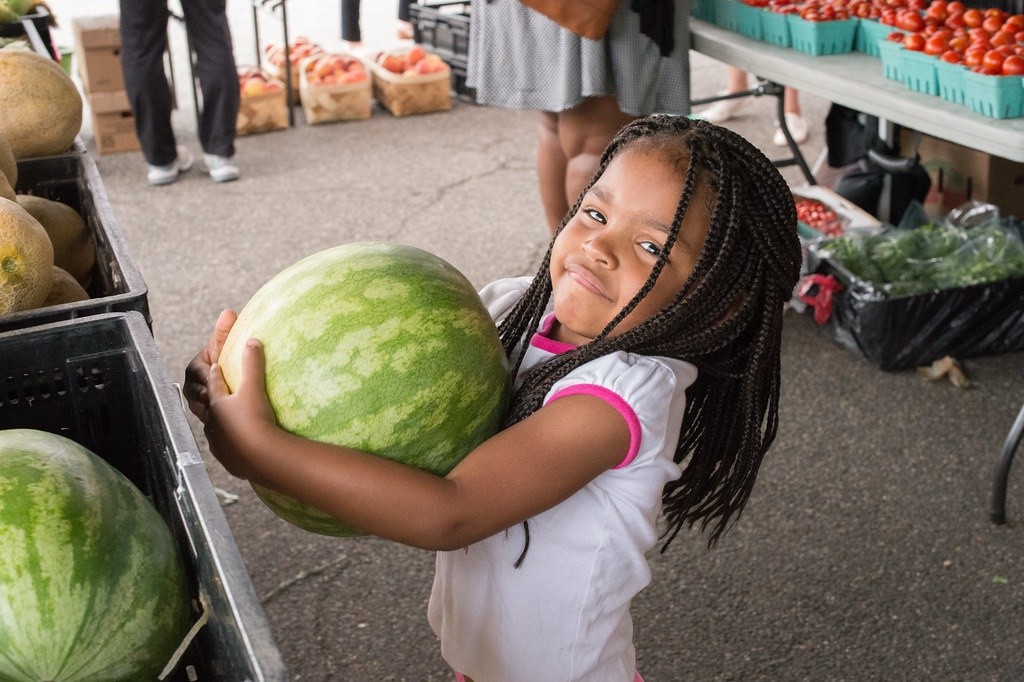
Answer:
[738,0,1024,76]
[796,198,845,237]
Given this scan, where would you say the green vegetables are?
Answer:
[812,218,1024,298]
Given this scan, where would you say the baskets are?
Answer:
[409,0,478,104]
[855,17,939,96]
[0,312,289,682]
[0,158,153,339]
[691,0,858,55]
[935,58,1024,120]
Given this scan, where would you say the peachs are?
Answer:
[237,35,449,100]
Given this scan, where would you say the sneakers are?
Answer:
[774,112,809,146]
[147,145,193,185]
[697,90,749,123]
[200,153,240,182]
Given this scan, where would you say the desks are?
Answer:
[676,19,1024,527]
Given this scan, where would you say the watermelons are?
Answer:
[0,427,192,682]
[212,241,513,538]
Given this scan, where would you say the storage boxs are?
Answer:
[787,183,885,275]
[808,219,1024,374]
[0,0,474,682]
[892,130,1024,227]
[692,0,1024,107]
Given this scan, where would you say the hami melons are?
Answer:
[0,46,96,317]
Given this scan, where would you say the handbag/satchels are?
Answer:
[519,0,619,41]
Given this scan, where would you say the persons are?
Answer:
[179,114,805,681]
[465,1,691,239]
[112,1,243,186]
